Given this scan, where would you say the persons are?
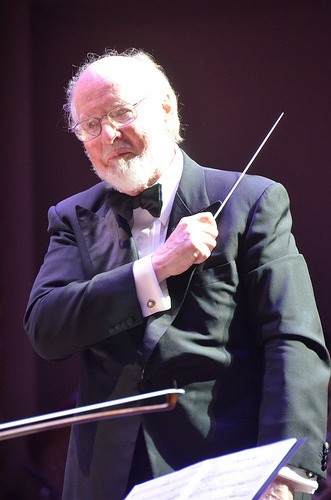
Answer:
[23,51,327,499]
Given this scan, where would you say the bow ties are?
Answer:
[109,183,162,227]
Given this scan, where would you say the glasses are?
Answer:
[69,99,146,141]
